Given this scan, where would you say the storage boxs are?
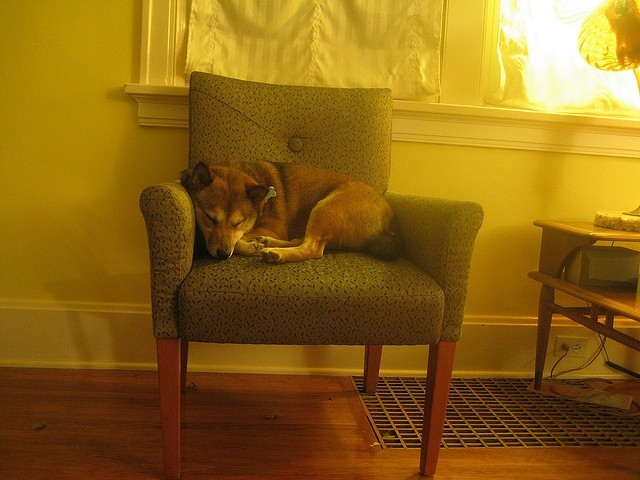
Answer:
[554,246,640,285]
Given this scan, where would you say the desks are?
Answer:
[527,219,640,390]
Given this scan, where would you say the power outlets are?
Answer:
[554,335,589,358]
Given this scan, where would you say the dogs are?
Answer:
[180,159,396,264]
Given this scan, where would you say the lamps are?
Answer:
[578,0,640,232]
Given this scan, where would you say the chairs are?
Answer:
[138,70,485,480]
